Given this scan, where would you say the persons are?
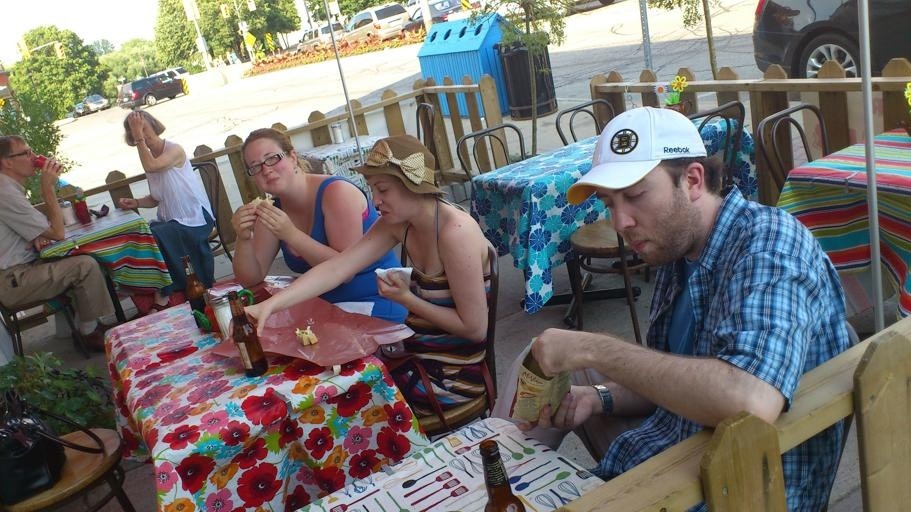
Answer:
[230,135,490,418]
[0,136,116,352]
[232,128,403,321]
[517,106,850,510]
[118,111,216,317]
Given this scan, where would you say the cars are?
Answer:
[84,95,112,113]
[71,102,86,119]
[402,1,485,35]
[497,0,615,23]
[150,67,190,83]
[406,1,420,12]
[752,1,911,80]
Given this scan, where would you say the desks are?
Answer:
[300,134,391,210]
[292,416,608,512]
[36,207,173,323]
[103,282,430,511]
[472,119,756,324]
[775,129,910,321]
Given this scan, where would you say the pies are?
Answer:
[248,196,273,208]
[293,324,318,346]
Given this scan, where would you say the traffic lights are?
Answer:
[220,3,231,19]
[53,42,66,60]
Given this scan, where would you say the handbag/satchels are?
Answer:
[0,389,105,507]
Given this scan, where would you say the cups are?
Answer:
[667,99,694,117]
[33,155,61,174]
[72,202,91,224]
[210,296,232,341]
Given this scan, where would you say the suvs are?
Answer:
[296,22,347,53]
[118,73,183,111]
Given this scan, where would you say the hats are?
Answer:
[349,135,449,194]
[566,107,708,206]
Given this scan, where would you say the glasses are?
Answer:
[5,148,32,158]
[247,151,289,176]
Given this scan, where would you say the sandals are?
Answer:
[140,301,173,318]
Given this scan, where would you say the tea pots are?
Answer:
[192,289,254,333]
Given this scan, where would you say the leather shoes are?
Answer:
[75,323,111,351]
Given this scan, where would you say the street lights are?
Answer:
[25,67,41,105]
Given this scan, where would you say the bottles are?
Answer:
[181,255,207,327]
[60,200,77,225]
[332,123,345,144]
[228,292,268,374]
[479,439,527,511]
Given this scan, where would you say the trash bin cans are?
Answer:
[417,11,525,119]
[493,31,558,121]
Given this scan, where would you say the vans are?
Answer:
[342,3,411,43]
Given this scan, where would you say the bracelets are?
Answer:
[592,385,613,418]
[135,138,144,143]
[135,199,139,208]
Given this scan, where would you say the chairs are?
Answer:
[456,123,525,183]
[410,100,473,204]
[756,103,828,193]
[687,99,746,182]
[564,218,654,347]
[555,97,615,146]
[0,250,126,361]
[164,161,235,282]
[400,232,500,436]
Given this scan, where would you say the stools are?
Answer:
[0,427,137,512]
